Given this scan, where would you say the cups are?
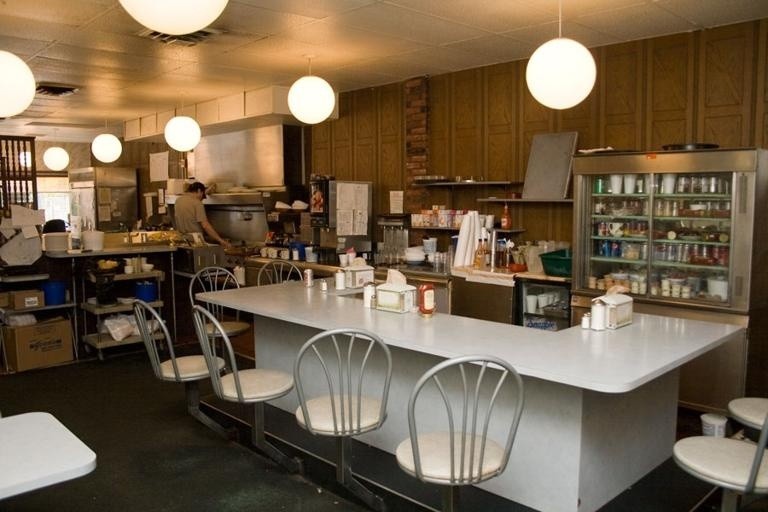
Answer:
[609,174,623,194]
[434,257,441,273]
[338,254,348,266]
[623,173,638,194]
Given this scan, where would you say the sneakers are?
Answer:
[173,336,186,346]
[187,334,200,346]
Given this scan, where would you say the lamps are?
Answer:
[43,128,70,171]
[118,0,229,36]
[160,108,200,152]
[287,58,336,127]
[90,117,123,164]
[525,0,597,110]
[0,50,36,118]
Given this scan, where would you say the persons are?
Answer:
[174,181,231,291]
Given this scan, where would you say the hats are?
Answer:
[185,181,209,200]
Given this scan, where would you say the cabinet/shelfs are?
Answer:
[517,283,570,329]
[73,266,167,357]
[571,152,767,314]
[411,177,521,261]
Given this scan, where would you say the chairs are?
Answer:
[188,265,251,373]
[292,328,393,512]
[396,355,526,511]
[129,300,227,436]
[188,306,297,469]
[728,396,768,439]
[671,412,768,512]
[257,259,302,286]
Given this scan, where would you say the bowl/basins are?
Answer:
[142,265,154,272]
[406,261,423,265]
[125,266,133,274]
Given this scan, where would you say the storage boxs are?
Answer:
[1,317,74,373]
[10,290,45,310]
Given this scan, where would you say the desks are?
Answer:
[196,275,744,512]
